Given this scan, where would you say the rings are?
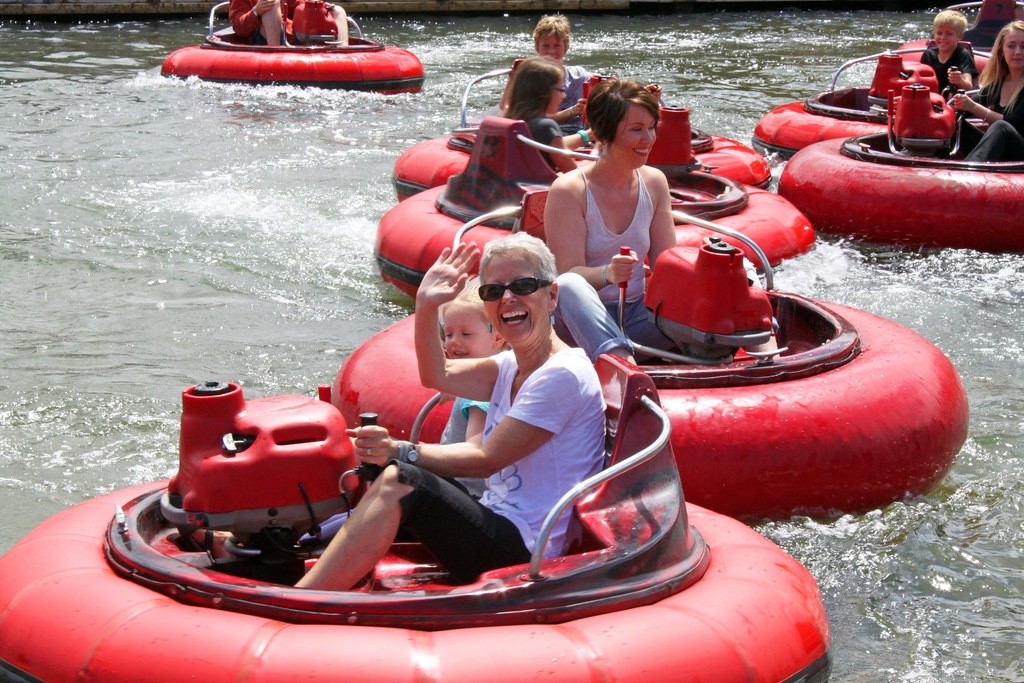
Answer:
[366,447,371,456]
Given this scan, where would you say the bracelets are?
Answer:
[252,6,261,19]
[397,439,409,462]
[602,264,609,287]
[983,109,989,121]
[577,129,590,146]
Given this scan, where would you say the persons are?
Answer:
[230,0,348,47]
[502,54,596,175]
[299,282,508,546]
[533,12,663,137]
[920,10,979,103]
[933,17,1024,162]
[543,77,780,366]
[293,230,607,592]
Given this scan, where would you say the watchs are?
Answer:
[407,443,419,463]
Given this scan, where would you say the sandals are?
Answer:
[177,525,213,553]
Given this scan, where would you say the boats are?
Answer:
[0,354,832,683]
[161,2,426,94]
[752,0,1024,253]
[332,188,969,527]
[375,117,817,300]
[391,57,772,204]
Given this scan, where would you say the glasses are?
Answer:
[552,86,567,96]
[479,276,552,301]
